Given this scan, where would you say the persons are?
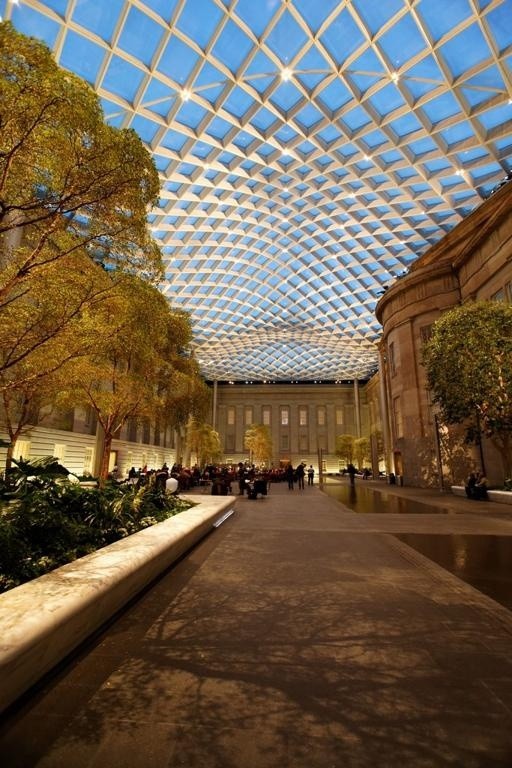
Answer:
[297,463,306,489]
[307,466,314,485]
[129,462,290,502]
[348,464,355,488]
[363,468,370,479]
[287,465,295,489]
[465,468,489,499]
